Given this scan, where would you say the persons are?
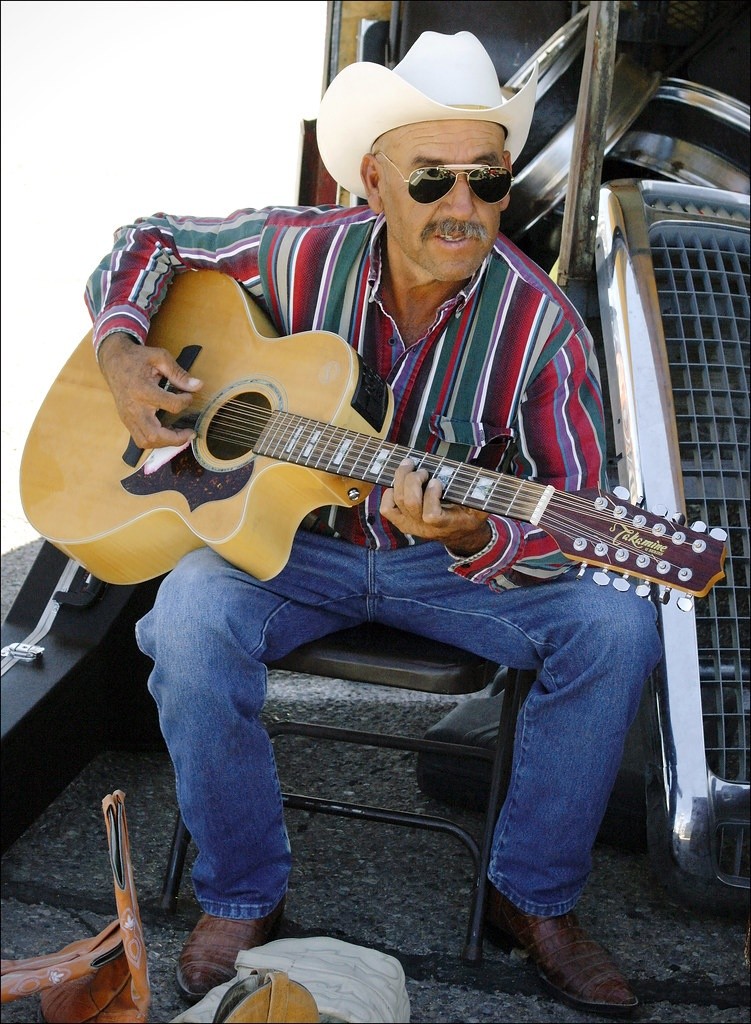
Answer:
[83,28,665,1012]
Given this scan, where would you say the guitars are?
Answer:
[11,262,735,617]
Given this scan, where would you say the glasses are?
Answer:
[373,148,515,204]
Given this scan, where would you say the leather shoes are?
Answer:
[175,894,286,1000]
[482,875,639,1013]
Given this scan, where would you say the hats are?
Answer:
[316,31,539,200]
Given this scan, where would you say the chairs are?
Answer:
[161,628,526,964]
